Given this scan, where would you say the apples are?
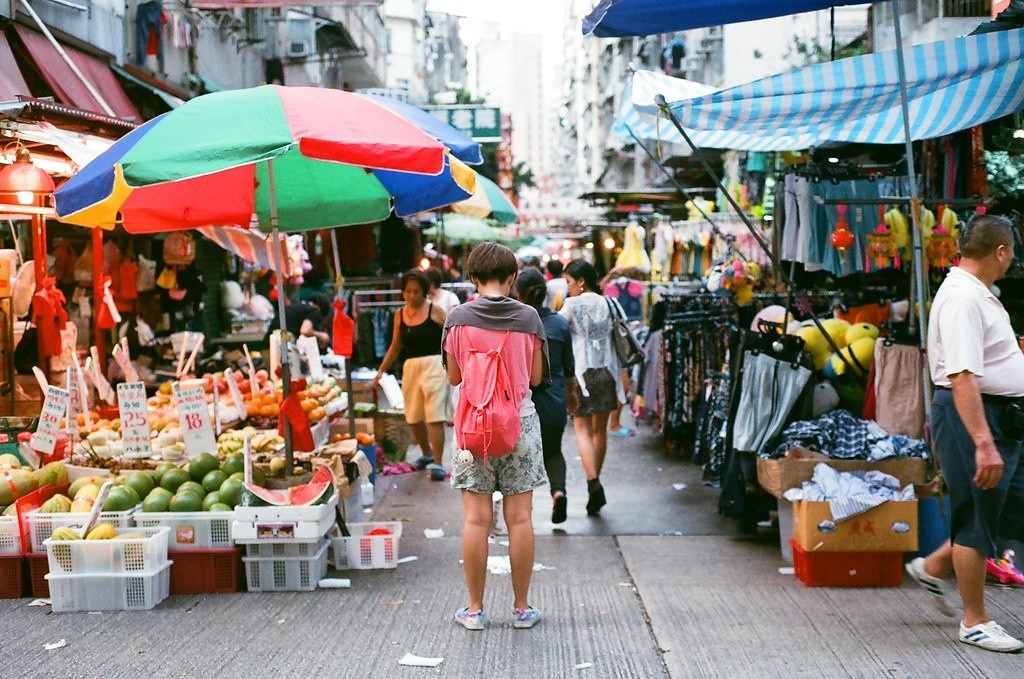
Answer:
[179,370,273,397]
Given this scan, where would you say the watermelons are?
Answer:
[98,451,265,512]
[240,464,334,506]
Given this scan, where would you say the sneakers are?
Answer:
[905,556,956,617]
[959,618,1024,652]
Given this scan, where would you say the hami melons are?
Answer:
[51,522,148,566]
[0,453,106,539]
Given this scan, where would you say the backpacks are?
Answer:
[617,278,645,325]
[454,326,520,464]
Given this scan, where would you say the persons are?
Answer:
[119,299,166,382]
[508,259,637,523]
[905,217,1024,650]
[424,266,462,425]
[370,269,450,477]
[441,243,551,630]
[260,284,335,369]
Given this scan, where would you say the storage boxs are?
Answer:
[336,380,375,404]
[0,415,404,613]
[757,456,927,588]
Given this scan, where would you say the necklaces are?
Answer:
[406,306,420,323]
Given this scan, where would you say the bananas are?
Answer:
[216,425,278,456]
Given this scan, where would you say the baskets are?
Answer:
[0,502,333,611]
[330,521,403,571]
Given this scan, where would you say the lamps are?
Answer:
[0,140,56,205]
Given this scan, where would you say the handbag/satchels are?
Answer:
[53,226,196,313]
[985,396,1024,441]
[12,290,42,376]
[605,294,646,367]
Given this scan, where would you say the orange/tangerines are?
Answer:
[333,432,375,445]
[242,390,328,420]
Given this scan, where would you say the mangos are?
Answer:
[58,382,235,453]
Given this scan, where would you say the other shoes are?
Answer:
[431,466,446,481]
[610,425,635,438]
[586,479,606,518]
[415,453,434,470]
[512,604,541,628]
[551,490,567,524]
[455,607,485,630]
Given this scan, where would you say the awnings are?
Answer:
[578,187,716,201]
[1,24,187,130]
[611,26,1023,419]
[582,0,885,39]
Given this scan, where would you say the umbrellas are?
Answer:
[434,175,518,246]
[331,93,484,278]
[53,84,478,478]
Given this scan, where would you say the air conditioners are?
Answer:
[286,39,308,58]
